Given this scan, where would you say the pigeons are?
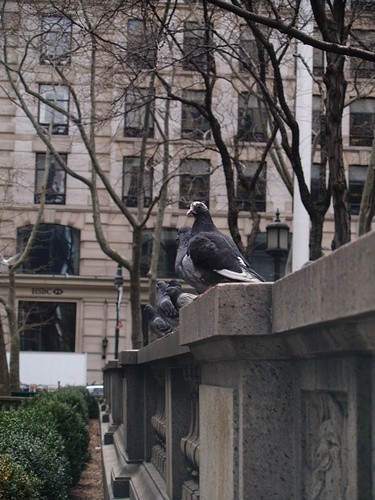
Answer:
[175,226,213,295]
[155,281,180,328]
[186,201,267,283]
[163,280,199,312]
[143,304,174,337]
